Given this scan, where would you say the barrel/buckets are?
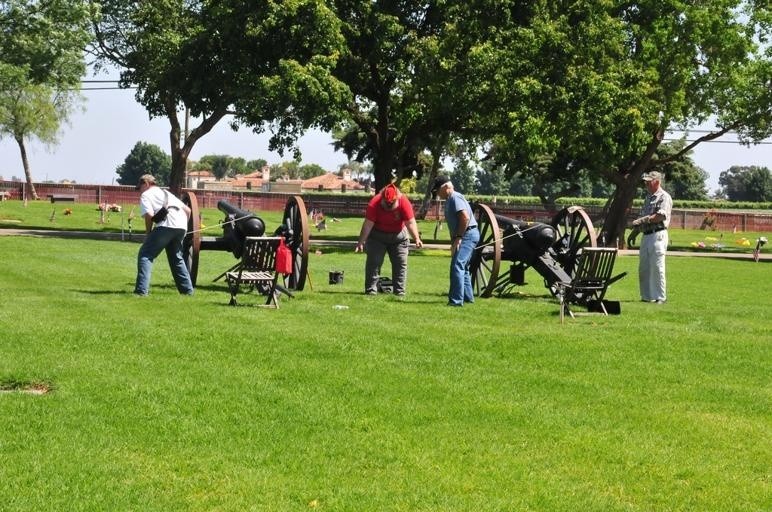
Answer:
[510,263,524,284]
[275,239,293,275]
[329,271,344,285]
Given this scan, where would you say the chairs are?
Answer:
[226,236,281,309]
[555,247,618,318]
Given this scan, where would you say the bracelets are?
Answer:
[455,235,465,239]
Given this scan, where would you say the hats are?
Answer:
[641,170,662,182]
[383,183,398,204]
[430,175,450,196]
[135,173,157,190]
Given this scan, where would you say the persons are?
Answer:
[431,175,480,308]
[626,171,673,305]
[131,174,194,296]
[352,182,423,299]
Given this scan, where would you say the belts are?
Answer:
[642,227,667,236]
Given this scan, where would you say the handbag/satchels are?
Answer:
[151,207,168,224]
